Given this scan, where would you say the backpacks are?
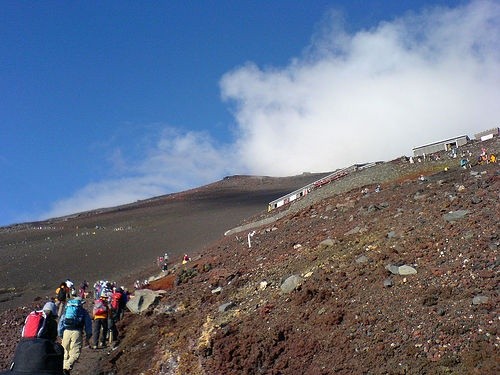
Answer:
[22,311,46,339]
[57,287,66,301]
[9,338,61,375]
[94,299,108,316]
[65,299,83,326]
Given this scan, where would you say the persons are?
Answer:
[58,298,93,375]
[55,282,69,316]
[461,156,468,169]
[376,184,381,192]
[182,253,189,264]
[92,281,127,349]
[21,302,58,340]
[429,153,440,161]
[135,280,140,289]
[66,279,74,298]
[478,153,496,165]
[12,317,64,375]
[454,150,473,158]
[409,153,426,163]
[361,187,368,195]
[79,280,88,299]
[163,253,168,270]
[419,175,425,182]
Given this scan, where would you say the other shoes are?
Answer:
[101,342,107,347]
[93,345,97,349]
[63,368,70,375]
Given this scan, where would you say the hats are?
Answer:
[100,293,109,298]
[74,297,85,303]
[43,302,57,313]
